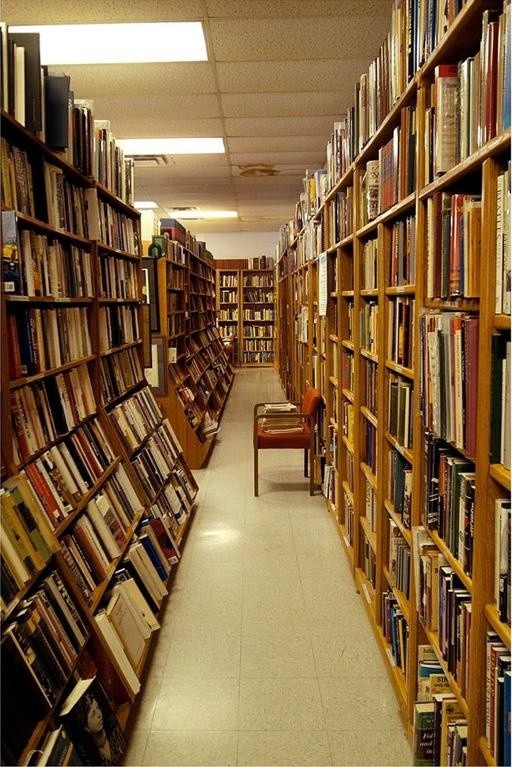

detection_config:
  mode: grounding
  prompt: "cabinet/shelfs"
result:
[277,0,512,766]
[142,236,235,468]
[0,107,199,763]
[239,268,276,367]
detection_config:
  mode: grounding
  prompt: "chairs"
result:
[252,387,322,497]
